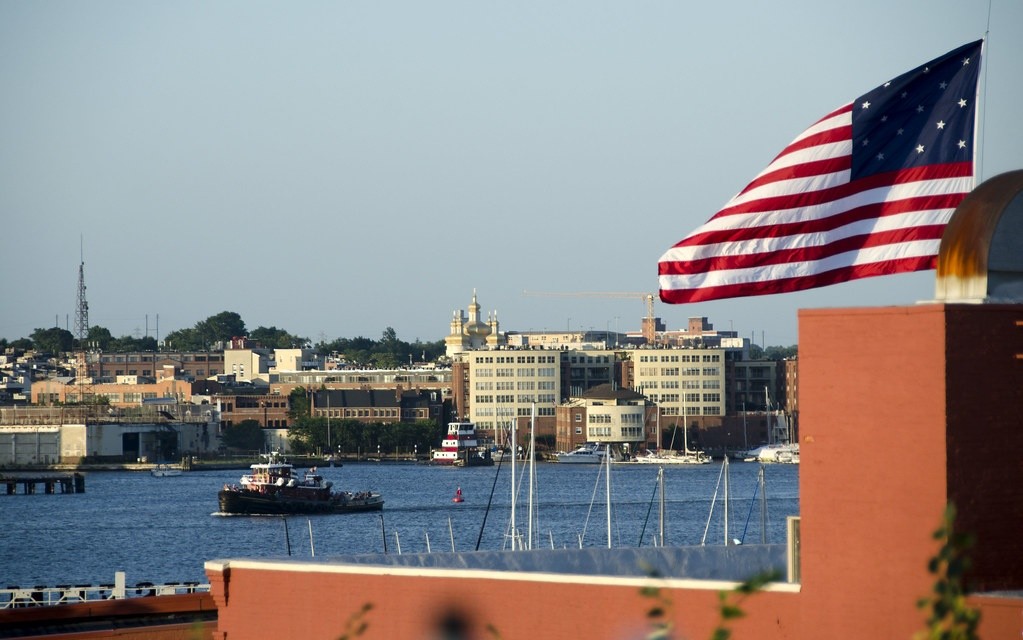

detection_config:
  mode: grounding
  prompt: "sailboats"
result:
[735,384,799,464]
[609,390,714,464]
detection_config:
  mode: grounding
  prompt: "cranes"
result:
[522,288,661,347]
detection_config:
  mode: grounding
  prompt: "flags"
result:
[658,37,985,303]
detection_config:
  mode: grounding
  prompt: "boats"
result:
[0,569,218,638]
[429,416,496,467]
[557,445,606,463]
[217,431,385,517]
